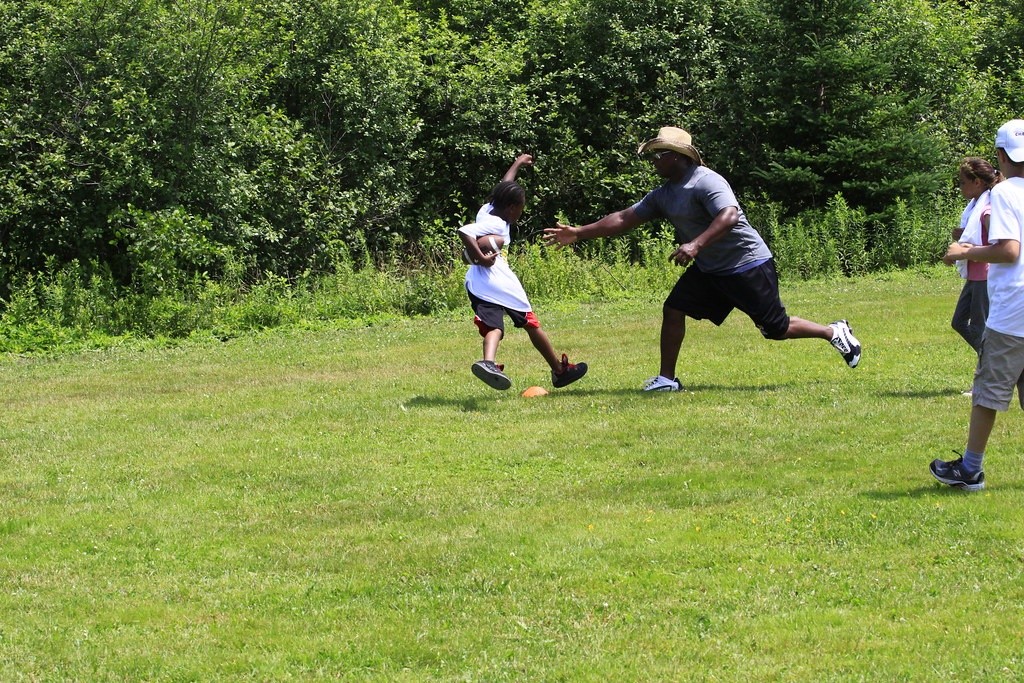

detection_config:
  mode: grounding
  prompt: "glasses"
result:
[994,148,999,155]
[653,149,671,160]
[960,178,973,184]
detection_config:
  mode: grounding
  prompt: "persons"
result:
[541,126,865,394]
[928,119,1024,497]
[456,151,587,391]
[944,156,1013,396]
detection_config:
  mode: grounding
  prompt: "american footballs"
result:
[461,234,504,266]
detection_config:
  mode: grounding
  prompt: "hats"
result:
[996,119,1024,162]
[638,126,706,167]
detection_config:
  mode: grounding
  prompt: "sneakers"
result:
[929,458,986,491]
[828,319,860,368]
[551,353,588,388]
[472,361,511,390]
[644,377,683,391]
